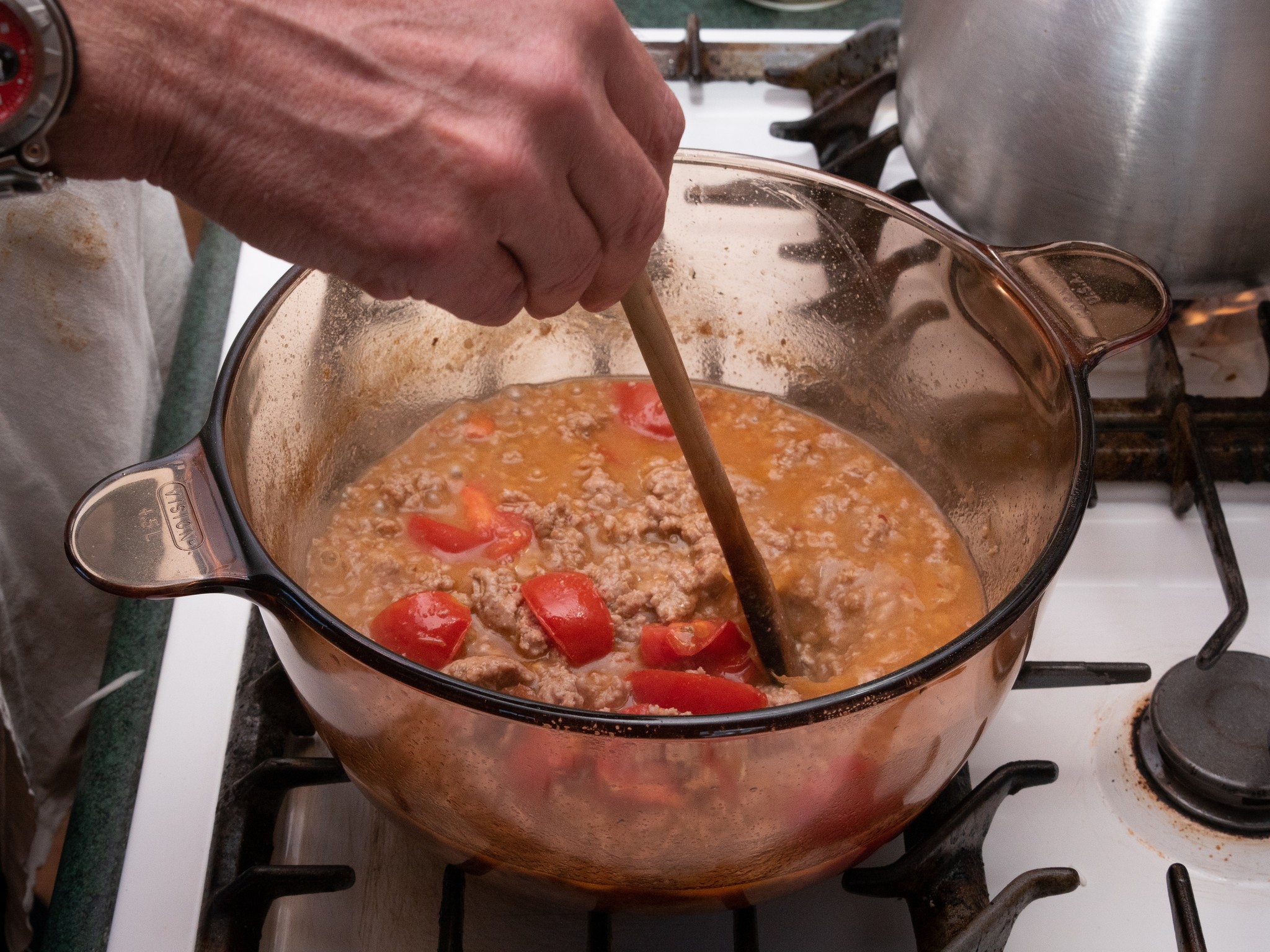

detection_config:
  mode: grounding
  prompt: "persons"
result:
[0,0,685,952]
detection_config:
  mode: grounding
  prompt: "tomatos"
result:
[371,488,901,847]
[616,383,677,437]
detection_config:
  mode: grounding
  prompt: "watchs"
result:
[0,0,81,199]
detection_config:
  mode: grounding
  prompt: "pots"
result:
[893,0,1270,306]
[65,147,1174,895]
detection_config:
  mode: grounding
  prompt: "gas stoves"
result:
[191,11,1270,952]
